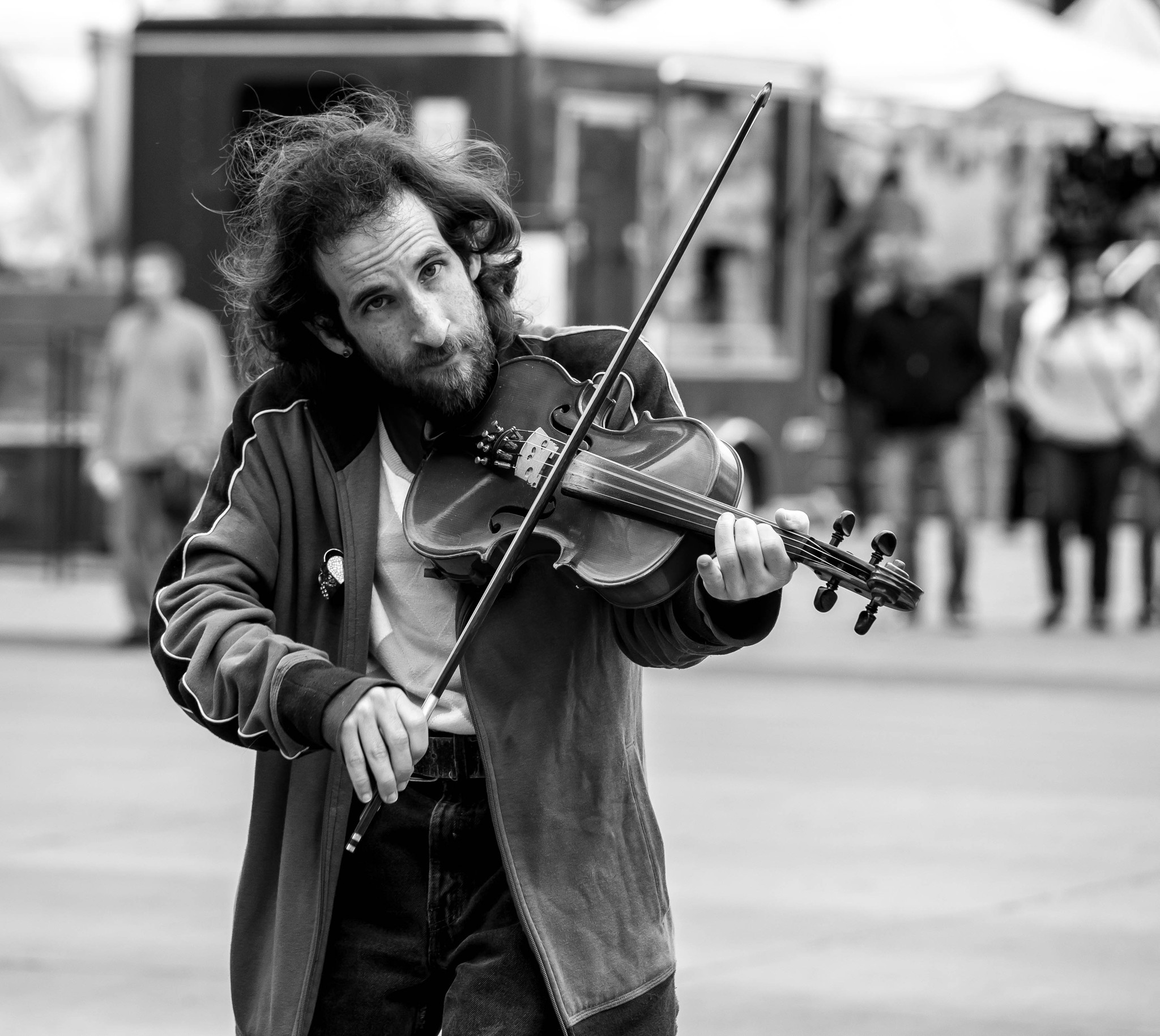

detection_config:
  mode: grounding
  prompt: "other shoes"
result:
[1033,600,1068,630]
[1087,603,1109,640]
[1135,600,1157,632]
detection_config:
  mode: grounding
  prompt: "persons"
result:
[805,165,1159,635]
[81,240,240,650]
[148,89,811,1036]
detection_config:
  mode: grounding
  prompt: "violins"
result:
[401,354,926,637]
